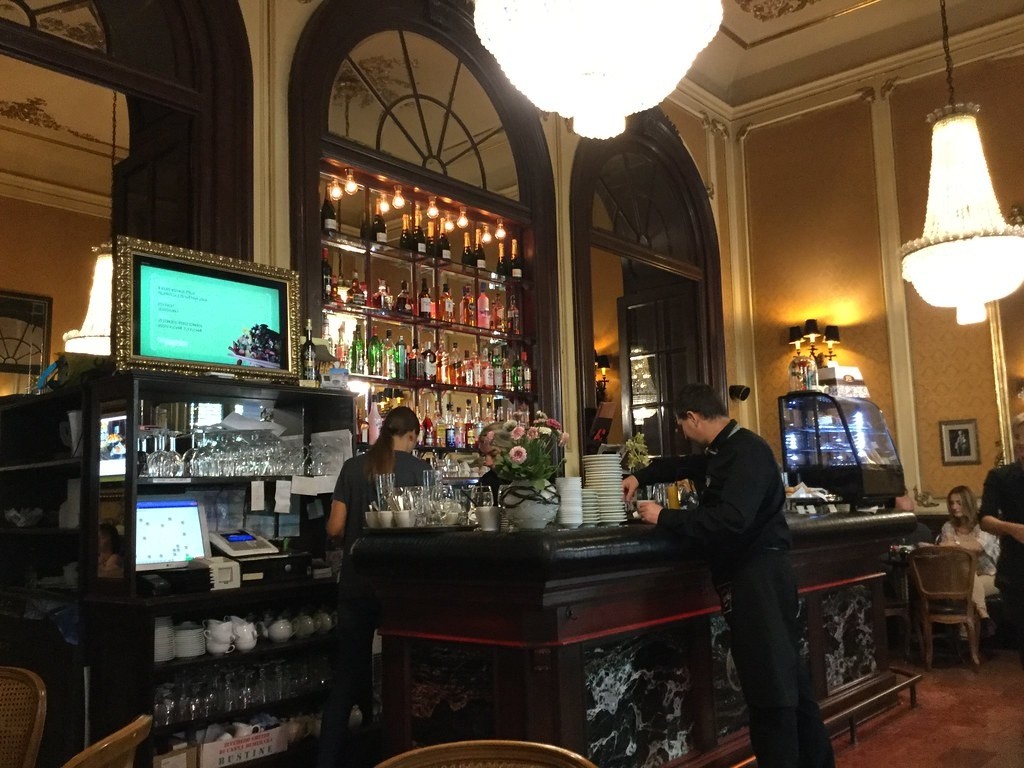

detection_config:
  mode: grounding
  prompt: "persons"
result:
[311,404,432,767]
[621,383,836,768]
[472,422,509,505]
[98,521,124,579]
[922,486,1002,664]
[979,413,1024,668]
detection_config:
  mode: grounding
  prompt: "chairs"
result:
[883,555,920,656]
[909,542,990,666]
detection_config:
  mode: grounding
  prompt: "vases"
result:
[501,479,561,529]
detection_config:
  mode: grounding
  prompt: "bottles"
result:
[302,180,531,448]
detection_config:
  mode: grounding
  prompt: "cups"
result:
[365,458,498,531]
[214,733,233,742]
[203,615,258,655]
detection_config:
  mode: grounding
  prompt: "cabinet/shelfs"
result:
[0,381,85,593]
[86,581,386,768]
[320,171,532,452]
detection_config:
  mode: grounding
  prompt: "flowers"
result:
[484,409,569,491]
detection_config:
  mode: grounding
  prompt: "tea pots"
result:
[256,610,339,643]
[276,705,362,744]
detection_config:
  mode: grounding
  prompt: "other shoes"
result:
[979,616,996,638]
[960,641,970,656]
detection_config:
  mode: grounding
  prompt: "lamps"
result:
[63,90,116,356]
[595,349,609,403]
[789,319,841,367]
[472,1,725,140]
[897,1,1024,325]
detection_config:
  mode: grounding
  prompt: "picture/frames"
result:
[939,418,981,465]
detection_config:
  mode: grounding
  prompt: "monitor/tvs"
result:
[134,494,212,571]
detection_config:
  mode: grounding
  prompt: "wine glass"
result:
[134,427,332,476]
[150,654,333,727]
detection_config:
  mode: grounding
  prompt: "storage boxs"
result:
[174,720,289,768]
[133,740,197,768]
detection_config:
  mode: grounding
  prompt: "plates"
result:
[154,615,205,663]
[554,453,628,528]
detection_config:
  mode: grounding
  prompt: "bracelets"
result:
[955,540,960,548]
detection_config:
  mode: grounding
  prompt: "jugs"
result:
[232,720,260,738]
